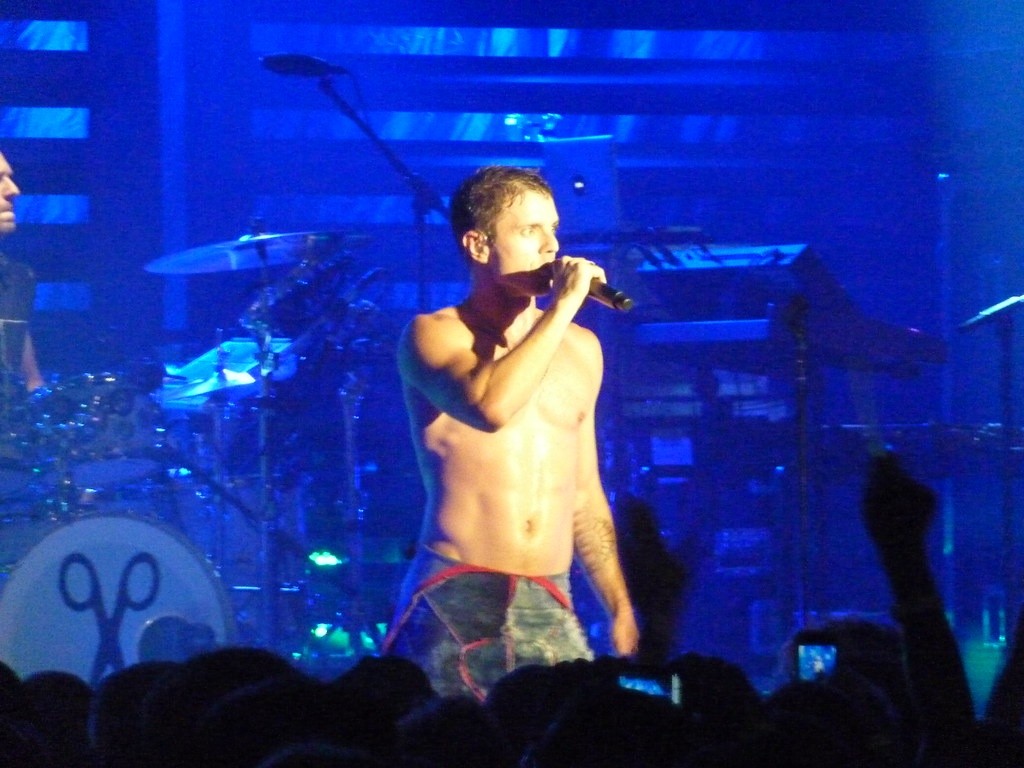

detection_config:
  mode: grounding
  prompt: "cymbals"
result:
[144,228,352,276]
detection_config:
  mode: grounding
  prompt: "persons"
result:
[0,450,1024,768]
[386,166,641,701]
[0,153,54,402]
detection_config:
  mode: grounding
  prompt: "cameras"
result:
[794,642,841,685]
[616,670,688,708]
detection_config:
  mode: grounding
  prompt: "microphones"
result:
[260,53,347,78]
[586,278,632,312]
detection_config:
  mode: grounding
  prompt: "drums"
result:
[0,507,238,688]
[176,472,307,593]
[0,366,33,499]
[21,371,167,494]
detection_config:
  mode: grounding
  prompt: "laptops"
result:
[542,135,701,232]
